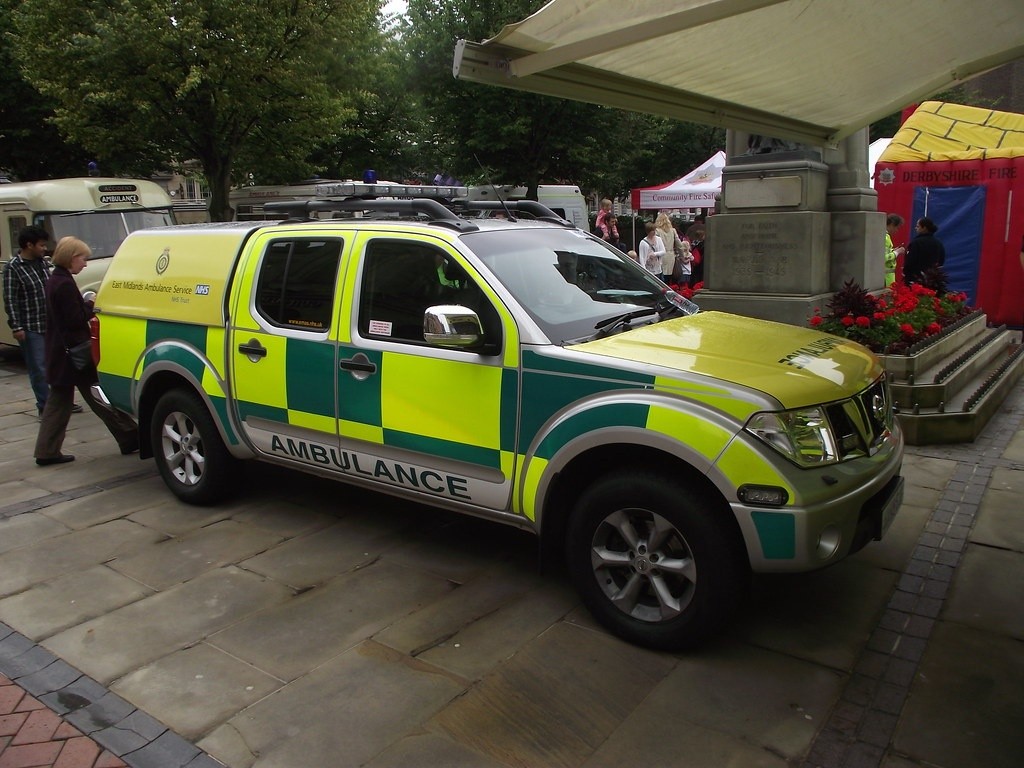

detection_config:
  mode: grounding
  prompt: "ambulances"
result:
[224,168,590,234]
[90,200,906,650]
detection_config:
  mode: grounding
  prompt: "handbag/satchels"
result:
[671,257,683,280]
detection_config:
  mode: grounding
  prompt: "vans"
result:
[1,177,177,356]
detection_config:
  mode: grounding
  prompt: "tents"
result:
[631,150,727,251]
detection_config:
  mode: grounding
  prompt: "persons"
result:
[3,226,84,423]
[902,218,945,288]
[495,211,514,219]
[594,198,619,250]
[34,236,138,466]
[654,213,706,289]
[627,222,666,282]
[885,214,905,287]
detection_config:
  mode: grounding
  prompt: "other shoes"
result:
[36,451,74,465]
[120,444,139,455]
[72,404,83,413]
[37,414,42,422]
